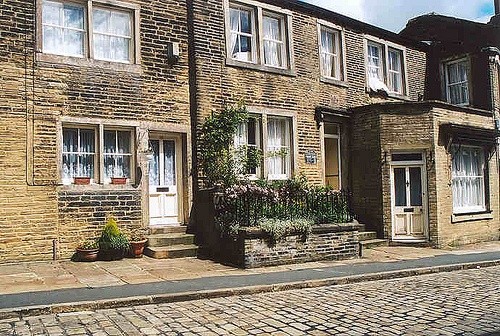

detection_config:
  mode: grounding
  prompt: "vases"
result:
[72,177,92,184]
[99,248,128,261]
[110,177,128,183]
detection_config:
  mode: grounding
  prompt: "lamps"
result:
[167,42,181,60]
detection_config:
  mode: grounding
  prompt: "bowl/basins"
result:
[111,177,127,184]
[73,177,90,185]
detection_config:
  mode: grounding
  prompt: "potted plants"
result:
[140,148,155,161]
[76,242,100,262]
[127,230,148,258]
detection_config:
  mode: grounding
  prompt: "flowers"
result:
[99,216,131,249]
